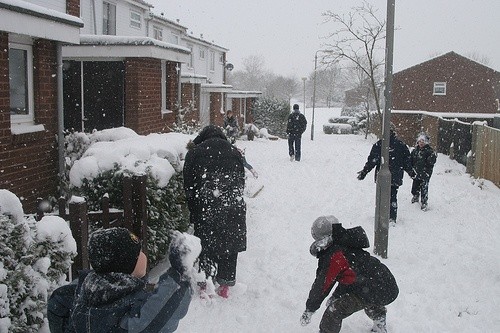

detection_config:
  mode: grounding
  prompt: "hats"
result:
[87,227,142,275]
[390,121,396,131]
[310,214,339,241]
[293,104,299,110]
[416,134,429,144]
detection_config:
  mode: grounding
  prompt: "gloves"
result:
[300,310,313,325]
[169,236,191,271]
[357,166,369,180]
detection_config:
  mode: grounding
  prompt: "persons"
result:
[242,156,258,179]
[64,227,194,333]
[183,125,247,298]
[356,122,417,228]
[285,104,307,162]
[223,110,238,143]
[410,132,438,211]
[299,215,399,333]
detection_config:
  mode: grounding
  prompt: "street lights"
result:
[301,77,308,117]
[311,49,335,141]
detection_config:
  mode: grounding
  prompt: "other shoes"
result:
[370,320,387,333]
[199,287,212,302]
[411,197,419,203]
[421,203,428,211]
[290,156,294,160]
[215,284,229,299]
